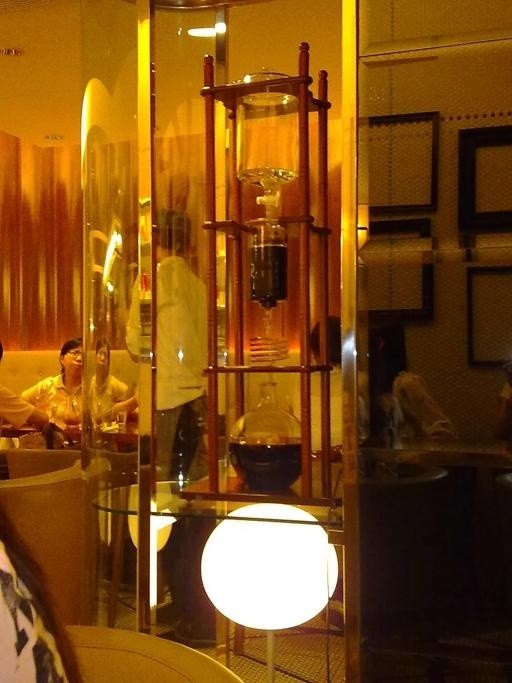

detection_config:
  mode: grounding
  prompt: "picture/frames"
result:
[356,109,439,216]
[466,264,511,367]
[359,218,433,324]
[457,125,511,232]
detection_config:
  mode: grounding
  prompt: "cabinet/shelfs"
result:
[180,38,339,505]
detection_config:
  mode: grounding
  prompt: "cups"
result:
[116,408,128,425]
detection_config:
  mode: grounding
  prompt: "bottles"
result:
[245,216,290,304]
[234,73,299,223]
[228,382,303,492]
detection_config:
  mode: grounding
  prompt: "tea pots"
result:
[63,394,82,428]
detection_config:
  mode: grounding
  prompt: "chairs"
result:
[2,424,245,480]
[2,459,105,672]
[64,622,246,681]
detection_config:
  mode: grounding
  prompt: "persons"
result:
[290,311,368,504]
[367,316,458,448]
[18,338,86,426]
[0,340,51,430]
[85,338,134,425]
[111,206,219,478]
